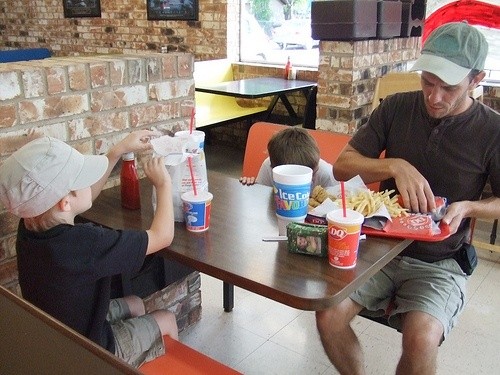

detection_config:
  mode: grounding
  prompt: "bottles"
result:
[285,57,292,80]
[120,152,140,210]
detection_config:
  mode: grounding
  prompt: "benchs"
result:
[242,120,386,193]
[0,286,245,375]
[192,59,267,129]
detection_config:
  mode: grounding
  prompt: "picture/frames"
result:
[147,0,198,20]
[63,0,101,18]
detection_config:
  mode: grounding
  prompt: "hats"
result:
[408,19,488,85]
[1,136,108,218]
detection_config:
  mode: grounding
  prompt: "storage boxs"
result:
[310,0,427,41]
[286,220,328,256]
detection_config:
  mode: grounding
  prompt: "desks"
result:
[195,77,317,126]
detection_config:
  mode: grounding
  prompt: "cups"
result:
[326,209,364,270]
[181,190,214,232]
[272,164,314,236]
[288,67,297,80]
[173,130,205,152]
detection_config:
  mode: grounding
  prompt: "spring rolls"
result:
[307,186,337,210]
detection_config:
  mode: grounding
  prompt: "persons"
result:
[316,20,500,375]
[239,128,341,204]
[0,136,178,370]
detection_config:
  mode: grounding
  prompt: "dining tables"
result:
[75,168,414,312]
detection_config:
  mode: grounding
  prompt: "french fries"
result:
[339,189,409,217]
